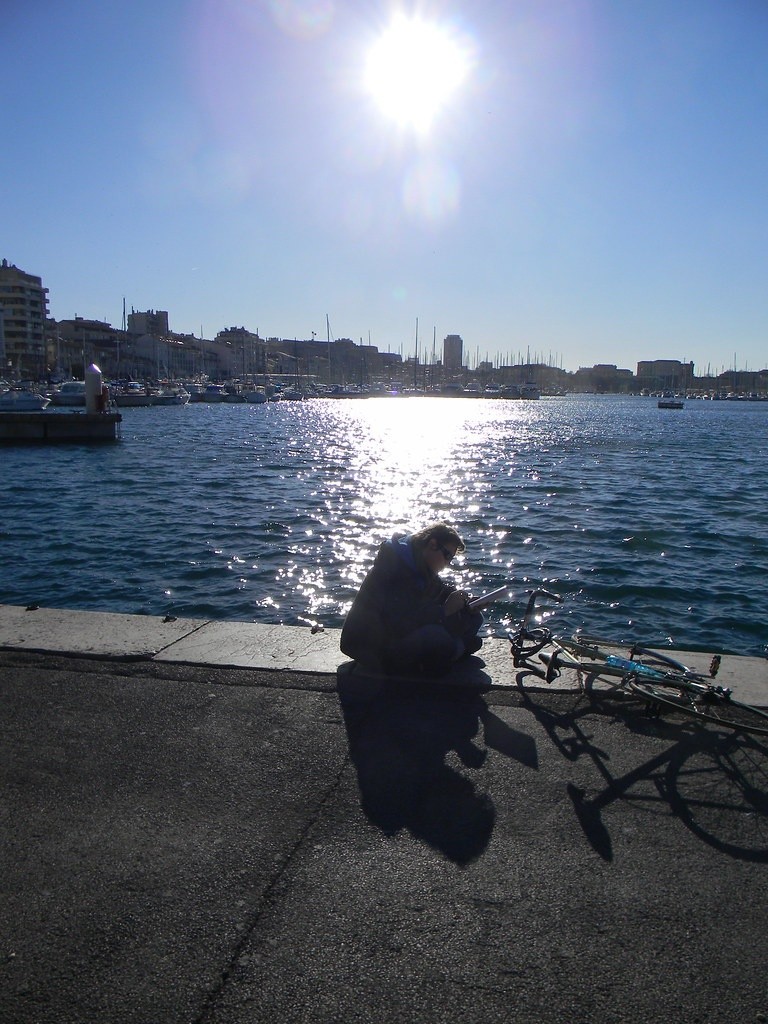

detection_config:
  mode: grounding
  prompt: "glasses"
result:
[437,543,454,562]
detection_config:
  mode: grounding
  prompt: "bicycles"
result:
[512,586,768,773]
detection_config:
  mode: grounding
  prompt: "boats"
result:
[54,388,191,406]
[188,383,303,405]
[640,388,768,401]
[544,388,567,397]
[0,388,51,411]
[463,382,541,400]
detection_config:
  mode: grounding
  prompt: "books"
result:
[468,586,509,610]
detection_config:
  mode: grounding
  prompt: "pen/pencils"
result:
[460,592,470,605]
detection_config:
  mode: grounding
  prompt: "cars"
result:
[276,379,441,393]
[1,373,243,390]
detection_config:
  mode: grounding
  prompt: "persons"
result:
[340,524,493,680]
